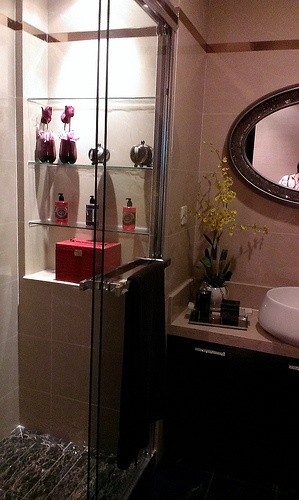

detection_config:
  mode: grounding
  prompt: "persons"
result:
[278,161,299,190]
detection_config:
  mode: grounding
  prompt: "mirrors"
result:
[225,81,299,210]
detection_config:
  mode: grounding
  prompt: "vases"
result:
[199,278,231,308]
[37,138,56,164]
[58,139,77,165]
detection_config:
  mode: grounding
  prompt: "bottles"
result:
[130,140,154,168]
[89,144,110,163]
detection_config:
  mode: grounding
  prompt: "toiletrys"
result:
[121,197,137,231]
[53,192,69,224]
[85,195,100,226]
[194,281,212,322]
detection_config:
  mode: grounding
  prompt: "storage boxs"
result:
[55,237,121,285]
[219,298,240,322]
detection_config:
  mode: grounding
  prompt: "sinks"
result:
[257,285,299,348]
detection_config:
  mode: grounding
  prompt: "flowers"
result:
[59,105,80,141]
[179,139,271,287]
[36,106,55,143]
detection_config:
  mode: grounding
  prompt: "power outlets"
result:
[180,205,188,226]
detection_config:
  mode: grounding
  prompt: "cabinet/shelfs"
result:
[167,335,299,486]
[27,96,156,237]
